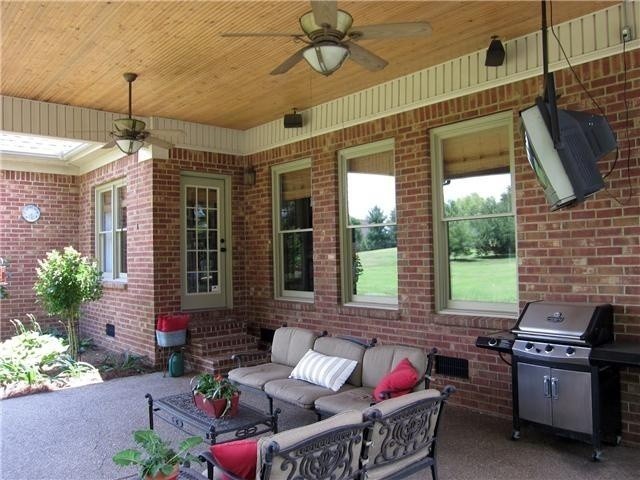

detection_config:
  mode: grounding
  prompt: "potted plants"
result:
[115,428,204,480]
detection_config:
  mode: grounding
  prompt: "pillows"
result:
[207,437,260,479]
[288,348,358,393]
[372,355,422,403]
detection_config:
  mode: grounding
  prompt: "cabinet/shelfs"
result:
[512,356,619,460]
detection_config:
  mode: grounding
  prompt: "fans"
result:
[70,73,186,149]
[223,0,433,74]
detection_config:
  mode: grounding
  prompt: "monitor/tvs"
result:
[519,96,618,213]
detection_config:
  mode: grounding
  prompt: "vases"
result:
[193,392,239,418]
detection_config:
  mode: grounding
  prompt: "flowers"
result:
[188,375,241,418]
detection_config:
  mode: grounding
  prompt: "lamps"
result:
[300,44,350,74]
[115,138,144,154]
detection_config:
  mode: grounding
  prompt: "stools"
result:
[161,346,194,377]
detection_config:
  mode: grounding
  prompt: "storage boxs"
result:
[155,329,187,347]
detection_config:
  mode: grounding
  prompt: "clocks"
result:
[22,205,40,222]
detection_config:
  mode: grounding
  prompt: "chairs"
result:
[315,338,440,425]
[226,322,328,411]
[359,384,455,479]
[265,330,381,432]
[200,410,369,479]
